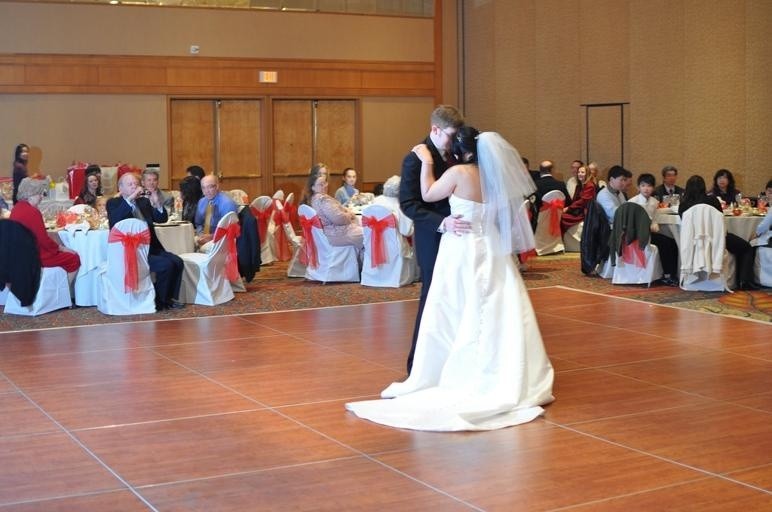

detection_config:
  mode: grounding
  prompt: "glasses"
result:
[314,181,329,186]
[433,122,456,142]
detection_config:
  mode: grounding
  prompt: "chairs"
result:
[297,204,361,284]
[611,202,663,285]
[284,193,295,223]
[362,204,421,287]
[223,205,256,293]
[563,221,583,252]
[535,190,565,255]
[275,200,307,280]
[91,218,158,316]
[0,222,71,313]
[179,211,238,305]
[752,245,772,291]
[680,203,736,292]
[265,187,284,261]
[247,195,273,266]
[590,201,621,279]
[509,200,532,270]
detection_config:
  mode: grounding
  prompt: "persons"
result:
[346,128,555,431]
[624,169,633,200]
[650,166,687,203]
[308,175,366,272]
[12,177,80,291]
[709,169,743,204]
[73,165,106,213]
[750,201,772,259]
[521,158,540,183]
[561,165,596,236]
[595,164,628,229]
[566,160,584,200]
[11,144,28,203]
[757,180,772,204]
[532,160,571,231]
[194,176,237,254]
[627,174,679,287]
[375,176,414,237]
[179,166,205,223]
[399,107,471,376]
[137,169,175,214]
[336,169,360,206]
[105,173,184,312]
[302,164,328,205]
[678,175,760,290]
[588,162,608,190]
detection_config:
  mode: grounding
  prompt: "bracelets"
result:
[422,161,436,166]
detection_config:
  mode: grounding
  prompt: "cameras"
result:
[140,188,152,196]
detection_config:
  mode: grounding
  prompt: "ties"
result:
[133,199,146,221]
[204,200,214,235]
